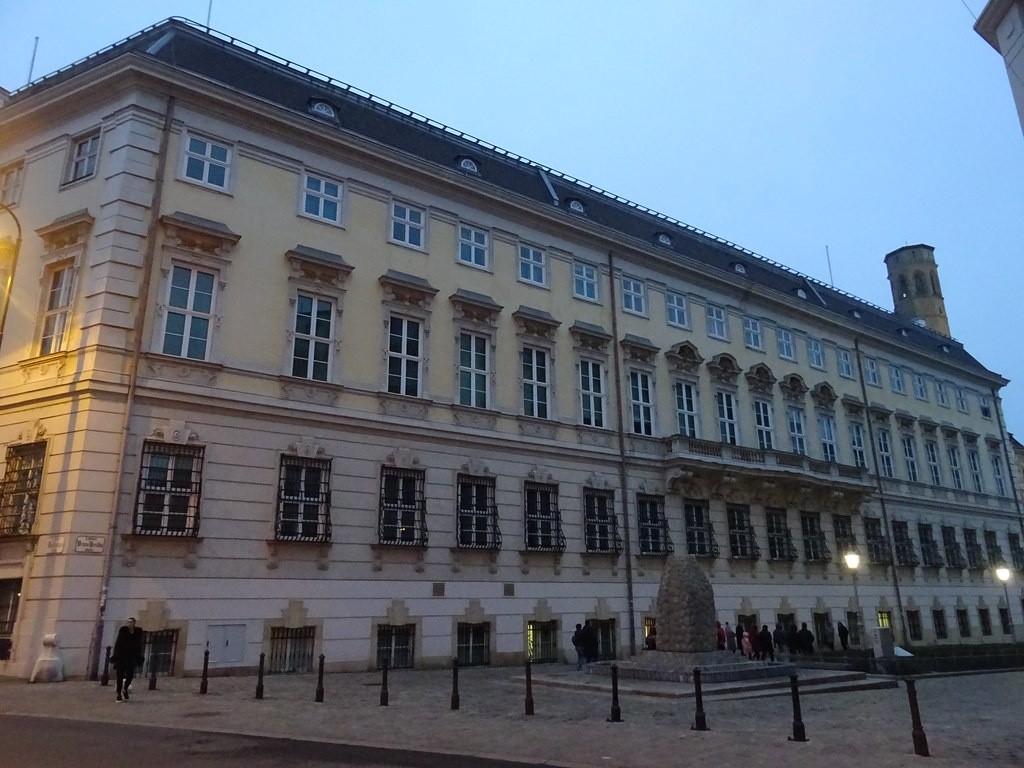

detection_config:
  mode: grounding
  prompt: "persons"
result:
[646,631,656,650]
[824,620,835,652]
[716,619,813,660]
[838,622,849,651]
[571,620,599,672]
[110,619,146,702]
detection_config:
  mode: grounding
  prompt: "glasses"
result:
[127,621,133,623]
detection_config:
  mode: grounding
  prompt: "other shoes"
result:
[116,694,123,703]
[122,689,129,700]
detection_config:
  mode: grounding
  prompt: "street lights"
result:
[996,563,1017,651]
[842,545,865,649]
